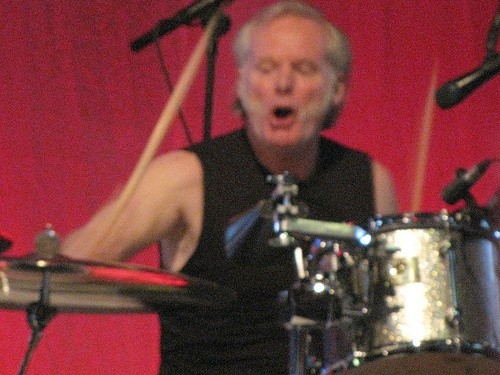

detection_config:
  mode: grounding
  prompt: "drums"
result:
[289,210,500,375]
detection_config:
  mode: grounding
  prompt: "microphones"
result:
[442,161,490,205]
[435,53,500,109]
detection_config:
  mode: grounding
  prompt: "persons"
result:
[61,1,399,375]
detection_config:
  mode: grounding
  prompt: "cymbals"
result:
[0,248,240,315]
